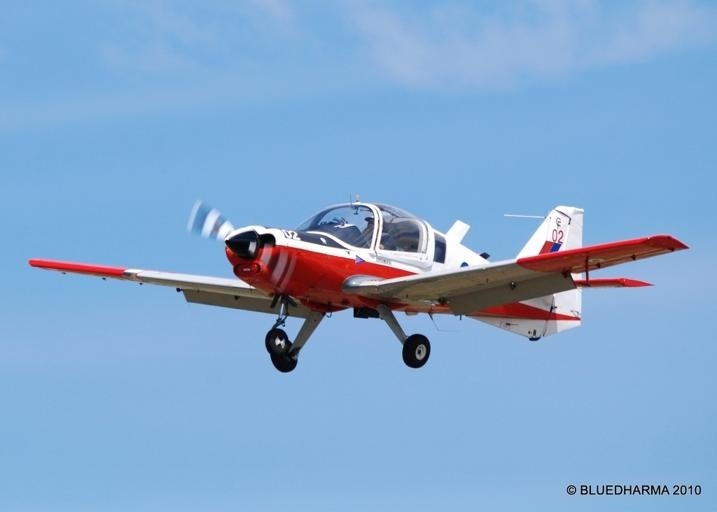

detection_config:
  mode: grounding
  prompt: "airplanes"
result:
[27,196,690,370]
